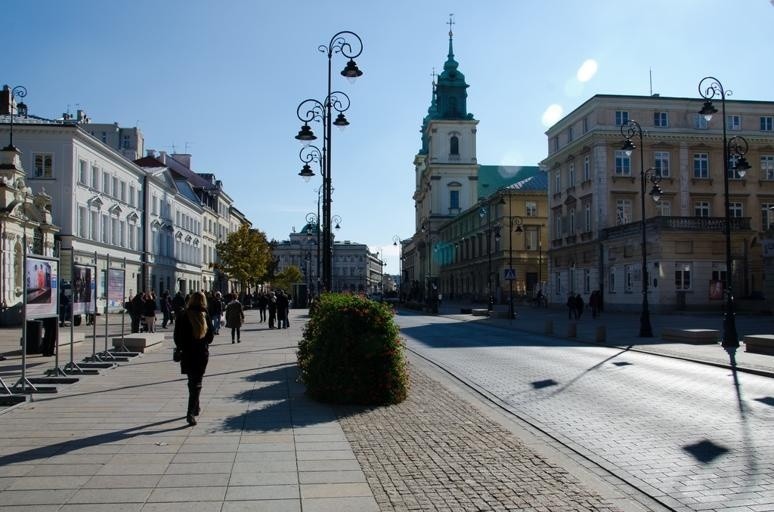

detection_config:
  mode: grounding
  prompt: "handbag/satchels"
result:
[174,347,182,362]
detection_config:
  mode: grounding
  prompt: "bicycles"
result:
[531,296,548,308]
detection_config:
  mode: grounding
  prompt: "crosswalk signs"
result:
[504,269,516,281]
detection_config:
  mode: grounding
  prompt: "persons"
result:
[567,288,601,320]
[126,288,291,344]
[173,290,214,426]
[27,263,45,288]
[60,288,70,327]
[536,289,544,307]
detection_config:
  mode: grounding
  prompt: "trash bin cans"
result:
[20,320,43,354]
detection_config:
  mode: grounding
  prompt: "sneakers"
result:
[186,414,198,425]
[194,408,200,416]
[232,339,241,344]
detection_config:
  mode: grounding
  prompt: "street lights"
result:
[697,76,753,366]
[495,186,523,320]
[392,234,406,308]
[294,91,352,306]
[478,200,500,313]
[421,219,438,314]
[620,119,664,339]
[376,248,386,302]
[295,29,364,307]
